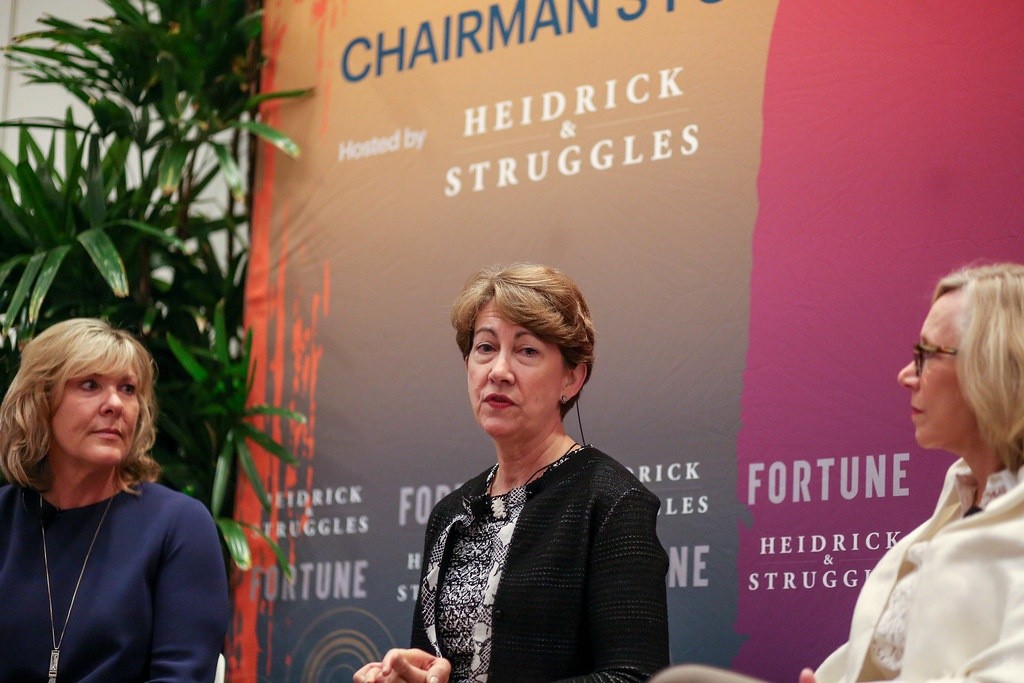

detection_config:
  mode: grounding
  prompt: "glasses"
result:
[913,344,958,375]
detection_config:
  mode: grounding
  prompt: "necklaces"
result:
[40,492,113,683]
[524,443,578,485]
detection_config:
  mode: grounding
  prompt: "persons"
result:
[353,263,669,683]
[800,262,1024,683]
[0,318,230,683]
